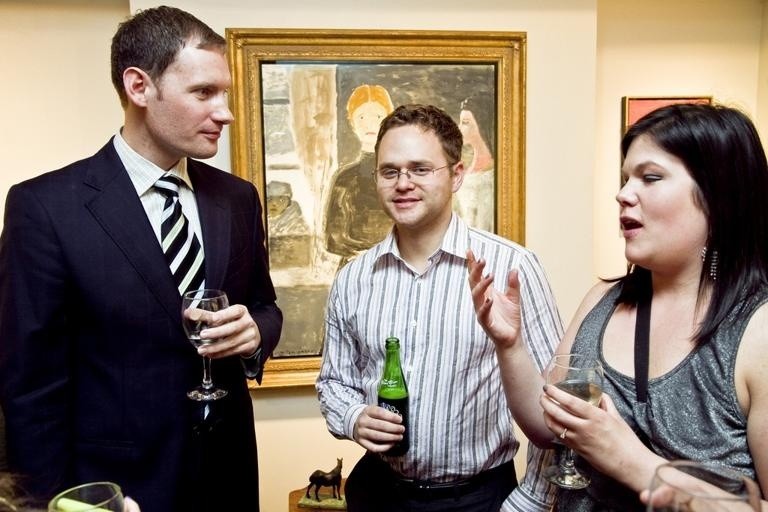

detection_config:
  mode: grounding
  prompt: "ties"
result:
[153,174,205,339]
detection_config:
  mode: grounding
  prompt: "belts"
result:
[367,456,510,496]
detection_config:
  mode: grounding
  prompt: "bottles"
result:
[377,337,410,456]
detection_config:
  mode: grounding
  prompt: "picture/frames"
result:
[224,26,528,390]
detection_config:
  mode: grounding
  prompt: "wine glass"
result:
[542,353,605,490]
[181,288,230,401]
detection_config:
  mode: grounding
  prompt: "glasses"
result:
[371,164,452,186]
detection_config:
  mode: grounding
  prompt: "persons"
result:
[0,5,283,512]
[450,91,495,232]
[324,84,394,263]
[265,180,308,269]
[465,103,768,512]
[316,104,565,512]
[640,481,758,512]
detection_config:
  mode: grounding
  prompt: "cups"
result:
[48,482,124,512]
[647,460,761,512]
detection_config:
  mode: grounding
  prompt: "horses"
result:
[306,457,343,502]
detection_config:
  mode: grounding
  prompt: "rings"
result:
[559,429,567,439]
